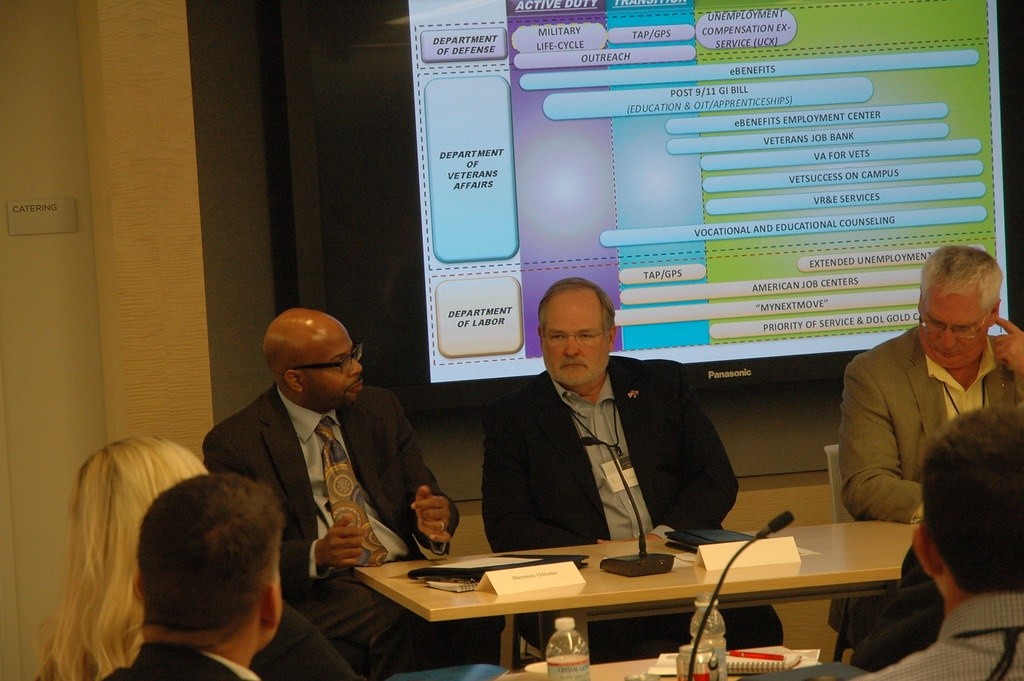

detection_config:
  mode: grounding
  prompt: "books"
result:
[403,552,590,592]
[643,643,824,673]
[656,526,759,556]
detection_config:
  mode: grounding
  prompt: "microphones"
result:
[688,510,794,681]
[580,436,676,576]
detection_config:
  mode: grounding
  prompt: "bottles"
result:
[691,594,727,681]
[546,617,591,681]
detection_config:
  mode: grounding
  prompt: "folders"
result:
[407,553,590,582]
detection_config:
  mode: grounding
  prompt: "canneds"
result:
[676,644,719,681]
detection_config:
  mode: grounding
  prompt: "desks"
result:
[354,519,919,661]
[589,645,822,680]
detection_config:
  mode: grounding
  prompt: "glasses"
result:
[920,298,994,339]
[543,330,609,347]
[285,342,363,374]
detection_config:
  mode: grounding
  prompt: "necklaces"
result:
[938,369,990,424]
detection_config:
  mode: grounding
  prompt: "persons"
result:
[480,276,784,664]
[204,305,517,680]
[27,433,361,681]
[99,472,291,681]
[846,405,1024,681]
[830,243,1024,649]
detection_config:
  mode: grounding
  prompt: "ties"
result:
[314,417,389,568]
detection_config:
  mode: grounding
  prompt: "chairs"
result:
[824,443,856,661]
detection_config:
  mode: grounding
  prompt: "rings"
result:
[438,520,446,531]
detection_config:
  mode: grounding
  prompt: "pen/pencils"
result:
[418,576,475,583]
[725,650,784,661]
[1000,362,1012,389]
